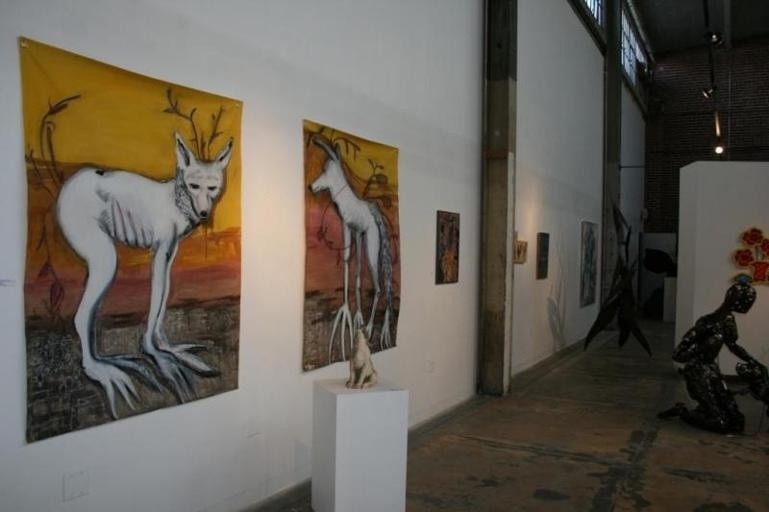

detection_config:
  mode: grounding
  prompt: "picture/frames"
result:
[536,232,550,280]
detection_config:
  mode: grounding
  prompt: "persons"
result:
[656,282,769,435]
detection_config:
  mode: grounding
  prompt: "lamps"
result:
[702,0,726,160]
